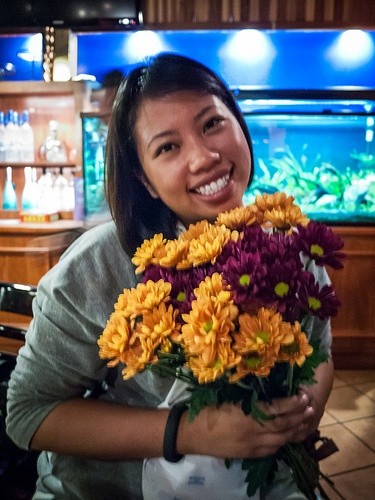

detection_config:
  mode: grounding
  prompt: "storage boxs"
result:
[78,88,375,232]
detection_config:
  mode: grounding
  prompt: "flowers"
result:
[97,191,348,499]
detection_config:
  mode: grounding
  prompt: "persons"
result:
[4,55,335,500]
[90,69,125,125]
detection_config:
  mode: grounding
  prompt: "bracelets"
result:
[163,404,189,463]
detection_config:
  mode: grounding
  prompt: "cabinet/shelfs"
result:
[0,80,91,356]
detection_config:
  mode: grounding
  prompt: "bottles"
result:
[3,166,18,210]
[39,120,67,164]
[21,167,77,214]
[0,109,35,164]
